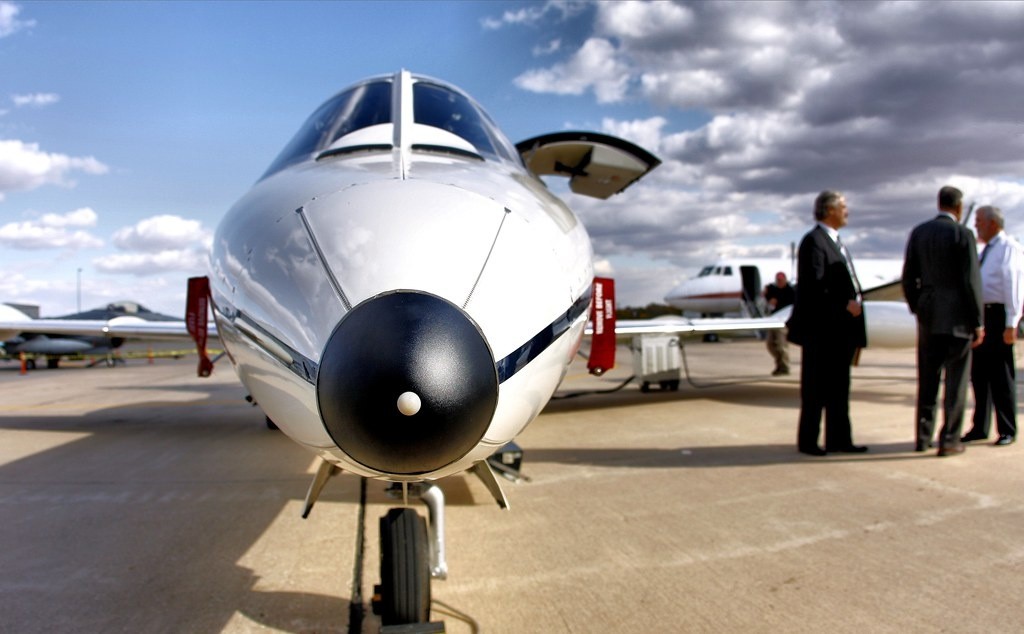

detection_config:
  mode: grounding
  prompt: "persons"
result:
[960,205,1024,446]
[764,273,795,375]
[902,186,985,456]
[791,189,869,456]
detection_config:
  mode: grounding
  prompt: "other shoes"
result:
[798,443,828,456]
[839,444,869,455]
[772,367,790,375]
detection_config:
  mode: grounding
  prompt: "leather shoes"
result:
[938,443,967,456]
[960,435,991,444]
[995,434,1014,446]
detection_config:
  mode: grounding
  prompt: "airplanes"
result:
[0,65,1023,634]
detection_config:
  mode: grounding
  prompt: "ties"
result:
[979,242,992,268]
